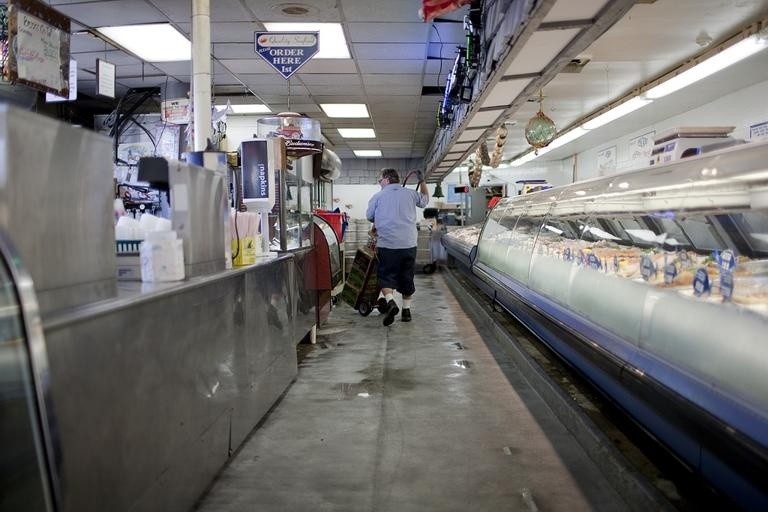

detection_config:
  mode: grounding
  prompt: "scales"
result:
[644,127,737,167]
[446,186,469,235]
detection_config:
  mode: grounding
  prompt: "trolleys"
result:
[341,171,422,317]
[415,224,437,274]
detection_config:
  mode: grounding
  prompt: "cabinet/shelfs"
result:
[269,138,318,256]
[470,132,767,469]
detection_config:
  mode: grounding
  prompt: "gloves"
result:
[416,169,424,182]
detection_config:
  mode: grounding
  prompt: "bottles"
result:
[139,229,186,282]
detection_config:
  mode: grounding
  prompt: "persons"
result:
[366,168,429,326]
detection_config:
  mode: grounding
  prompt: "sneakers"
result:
[402,309,411,322]
[383,300,399,326]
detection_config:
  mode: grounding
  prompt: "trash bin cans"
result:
[317,213,346,241]
